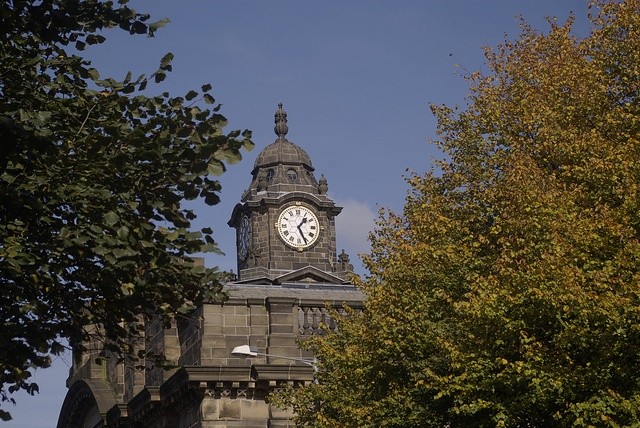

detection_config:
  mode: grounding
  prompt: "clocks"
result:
[237,216,251,262]
[274,200,325,252]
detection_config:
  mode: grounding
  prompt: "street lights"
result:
[230,343,319,412]
